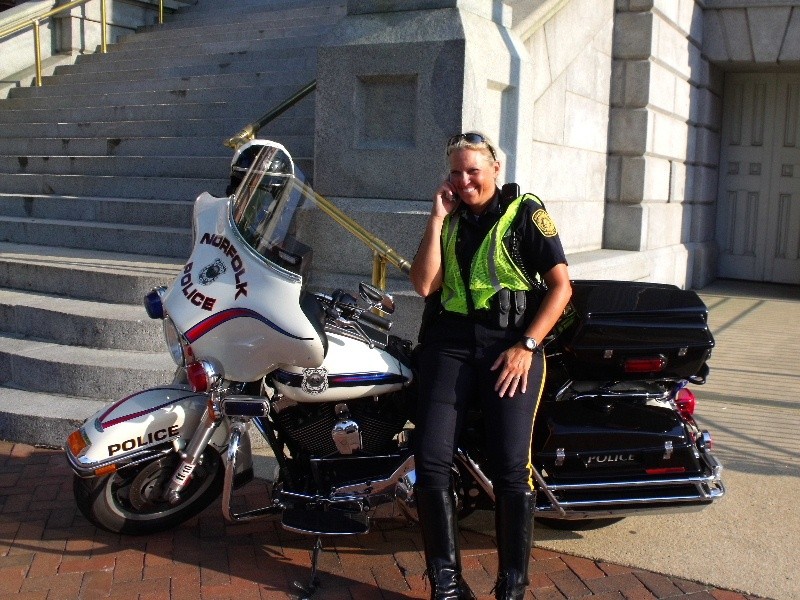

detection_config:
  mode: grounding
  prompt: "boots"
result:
[413,487,477,600]
[490,491,536,600]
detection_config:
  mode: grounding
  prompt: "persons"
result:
[410,130,572,600]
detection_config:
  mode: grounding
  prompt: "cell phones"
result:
[452,193,460,204]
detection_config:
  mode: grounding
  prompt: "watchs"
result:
[520,336,537,353]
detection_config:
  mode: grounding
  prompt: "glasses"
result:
[448,133,496,161]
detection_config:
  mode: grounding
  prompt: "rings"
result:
[515,378,520,379]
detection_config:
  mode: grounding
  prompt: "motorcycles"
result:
[62,138,726,600]
